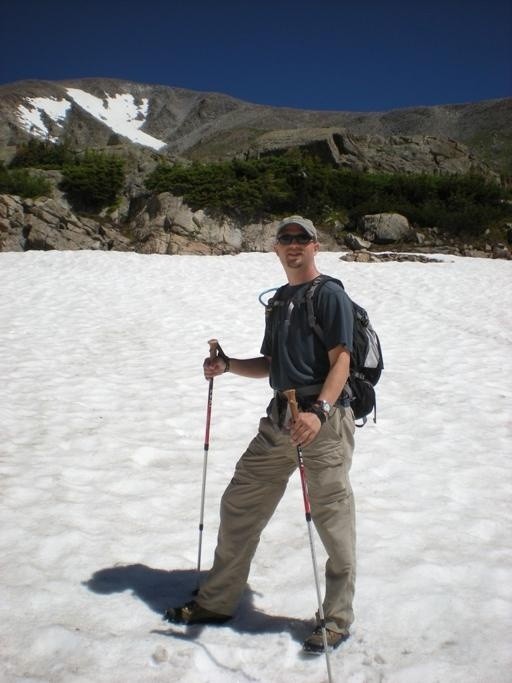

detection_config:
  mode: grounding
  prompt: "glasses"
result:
[279,233,312,245]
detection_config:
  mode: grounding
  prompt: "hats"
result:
[275,214,317,241]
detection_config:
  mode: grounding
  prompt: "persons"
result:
[160,213,359,651]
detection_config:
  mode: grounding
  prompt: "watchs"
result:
[310,396,332,415]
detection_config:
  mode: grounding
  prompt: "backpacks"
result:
[304,274,384,428]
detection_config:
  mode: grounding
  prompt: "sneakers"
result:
[165,599,233,625]
[303,625,344,655]
